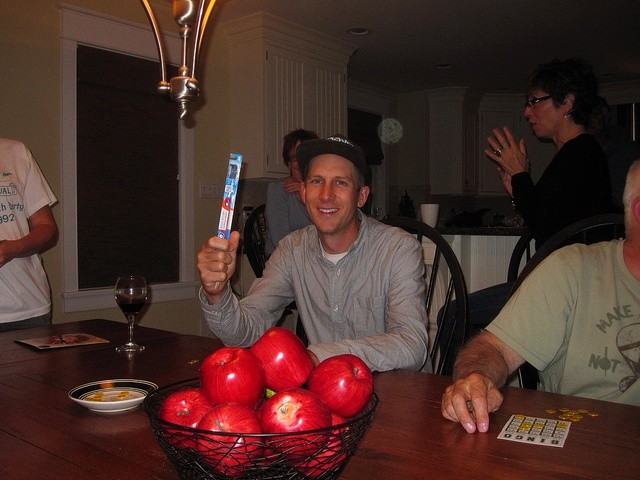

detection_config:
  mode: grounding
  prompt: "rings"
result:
[494,148,502,157]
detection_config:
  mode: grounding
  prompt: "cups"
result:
[420,204,440,228]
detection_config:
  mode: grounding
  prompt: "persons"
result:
[0,138,60,333]
[441,158,640,433]
[196,133,433,373]
[586,96,638,210]
[437,59,613,390]
[264,128,322,348]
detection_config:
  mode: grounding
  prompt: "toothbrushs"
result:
[218,163,239,239]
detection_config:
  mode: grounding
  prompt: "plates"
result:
[68,378,160,413]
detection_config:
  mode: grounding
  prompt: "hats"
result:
[297,134,368,179]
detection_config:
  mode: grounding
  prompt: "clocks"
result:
[377,118,405,145]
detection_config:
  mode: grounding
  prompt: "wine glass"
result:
[114,273,148,353]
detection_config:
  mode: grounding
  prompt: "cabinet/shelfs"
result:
[428,102,479,196]
[191,45,350,183]
[413,233,534,323]
[479,100,556,196]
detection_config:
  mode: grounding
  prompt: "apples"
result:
[195,400,263,475]
[259,387,333,460]
[251,407,281,465]
[157,386,213,449]
[285,413,351,476]
[199,346,267,411]
[309,354,373,418]
[249,326,315,393]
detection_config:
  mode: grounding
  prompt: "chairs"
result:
[296,216,470,377]
[507,224,543,284]
[505,224,630,390]
[244,203,271,281]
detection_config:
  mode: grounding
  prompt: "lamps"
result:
[139,1,221,124]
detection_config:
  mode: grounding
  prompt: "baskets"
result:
[143,377,379,479]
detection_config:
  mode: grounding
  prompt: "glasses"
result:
[524,94,556,108]
[288,154,297,161]
